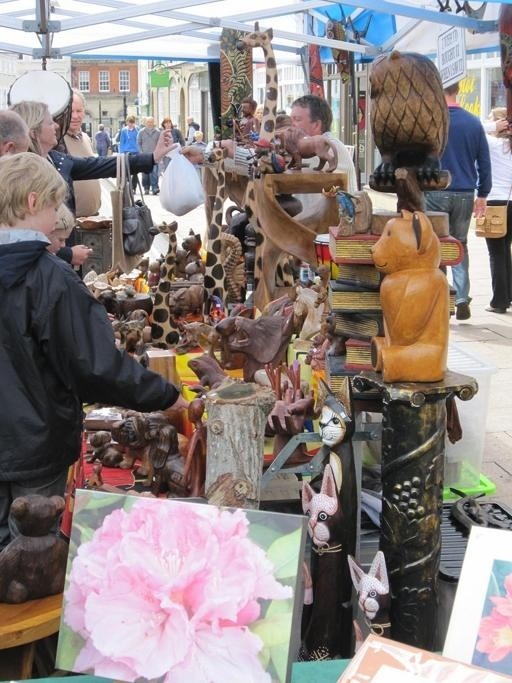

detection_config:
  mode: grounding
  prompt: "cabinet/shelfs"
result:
[76,227,112,279]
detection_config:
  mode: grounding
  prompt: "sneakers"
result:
[144,187,161,195]
[454,300,471,320]
[485,305,506,314]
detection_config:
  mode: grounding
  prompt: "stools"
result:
[0,591,63,679]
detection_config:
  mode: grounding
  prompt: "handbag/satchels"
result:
[121,149,155,256]
[474,204,509,239]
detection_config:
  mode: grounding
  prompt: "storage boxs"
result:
[361,340,500,489]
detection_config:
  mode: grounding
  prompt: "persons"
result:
[474,106,512,314]
[94,114,200,196]
[11,101,178,279]
[2,111,29,155]
[0,154,188,549]
[185,398,207,498]
[425,80,492,319]
[45,202,74,255]
[237,99,260,145]
[56,92,101,217]
[179,95,358,222]
